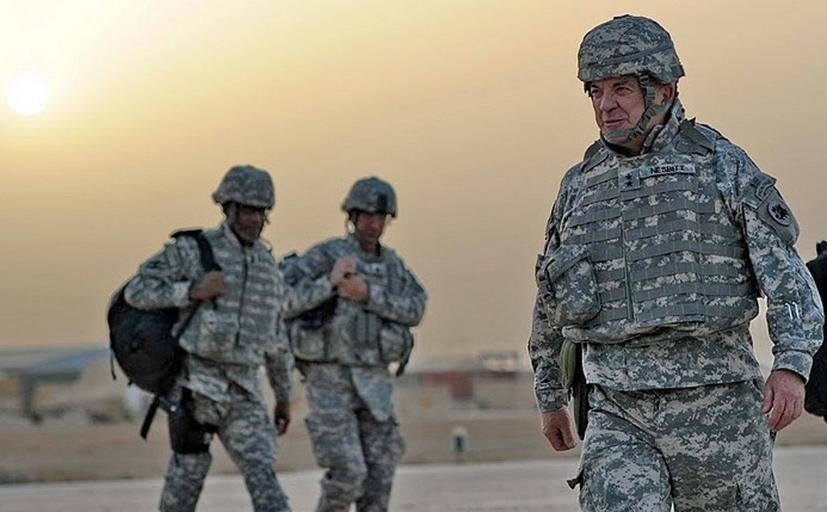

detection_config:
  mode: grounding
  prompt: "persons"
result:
[279,176,427,512]
[124,164,295,512]
[527,14,826,512]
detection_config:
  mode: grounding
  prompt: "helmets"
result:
[213,166,274,212]
[341,176,398,218]
[578,15,684,85]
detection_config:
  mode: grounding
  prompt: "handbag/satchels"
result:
[107,230,218,400]
[799,240,827,424]
